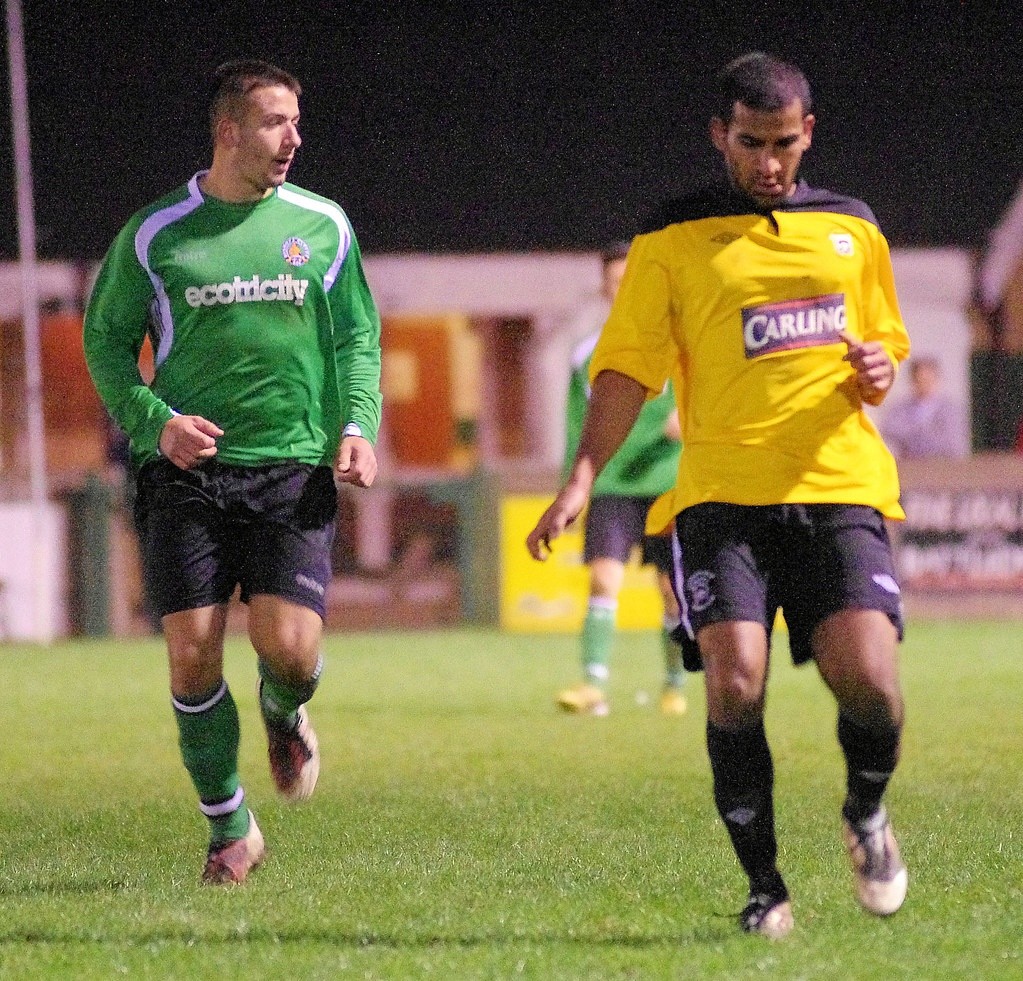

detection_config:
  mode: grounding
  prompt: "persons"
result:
[878,356,970,459]
[554,240,684,714]
[528,53,909,932]
[83,60,382,885]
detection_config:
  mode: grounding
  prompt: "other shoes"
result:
[660,688,686,713]
[557,682,609,716]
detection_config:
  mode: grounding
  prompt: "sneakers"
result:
[712,894,796,938]
[202,809,266,885]
[840,801,909,915]
[256,677,320,801]
